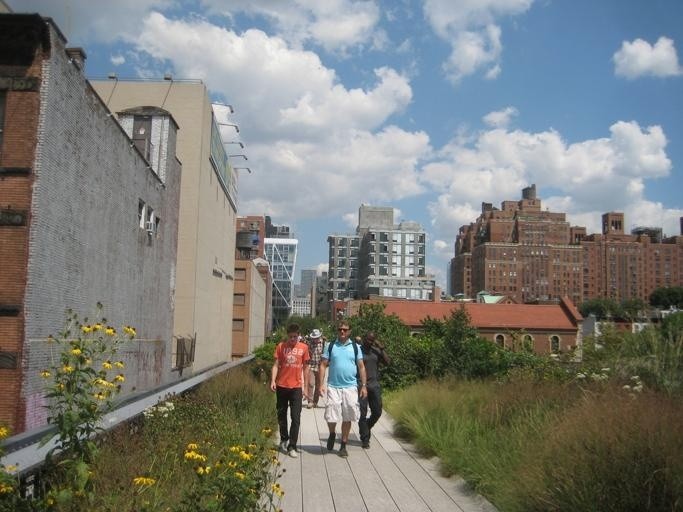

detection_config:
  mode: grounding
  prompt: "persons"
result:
[318,319,369,456]
[268,324,310,456]
[355,332,390,448]
[305,328,325,408]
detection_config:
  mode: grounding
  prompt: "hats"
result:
[310,329,322,339]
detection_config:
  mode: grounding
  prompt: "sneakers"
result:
[327,433,336,450]
[280,441,297,458]
[340,448,347,456]
[307,401,318,408]
[362,441,370,449]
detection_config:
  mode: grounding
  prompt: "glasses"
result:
[337,328,350,332]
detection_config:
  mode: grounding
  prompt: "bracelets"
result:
[380,348,384,350]
[360,383,367,387]
